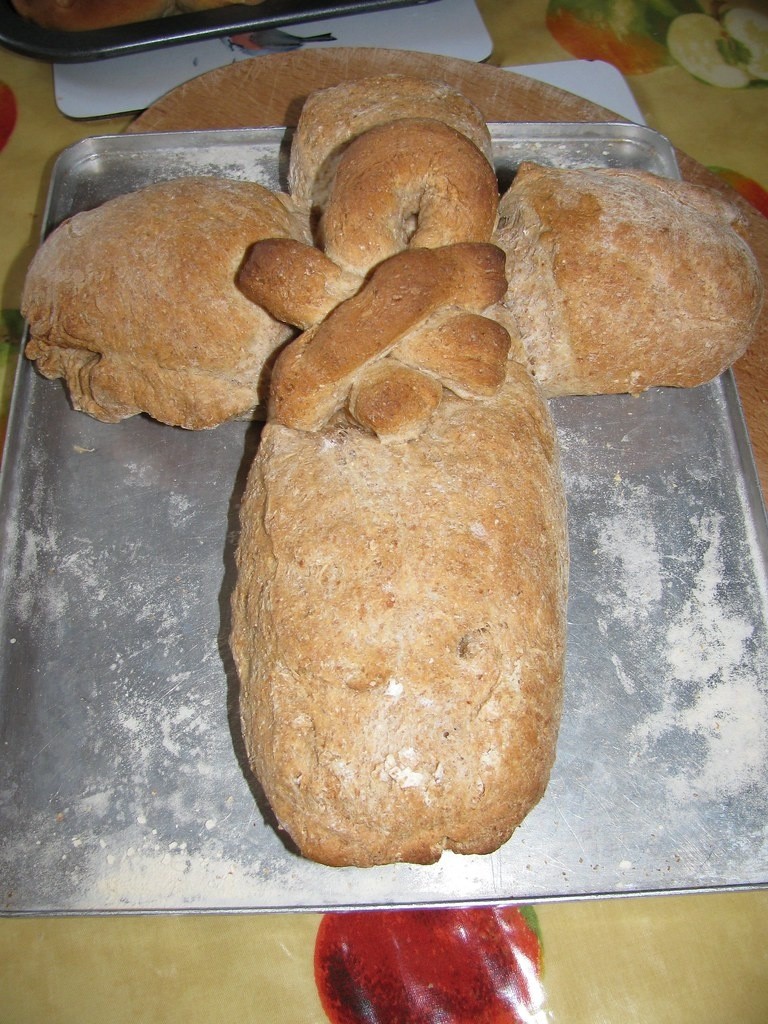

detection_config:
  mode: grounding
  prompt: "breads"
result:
[19,73,764,866]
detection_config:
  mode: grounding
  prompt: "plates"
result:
[0,122,768,915]
[0,0,441,64]
